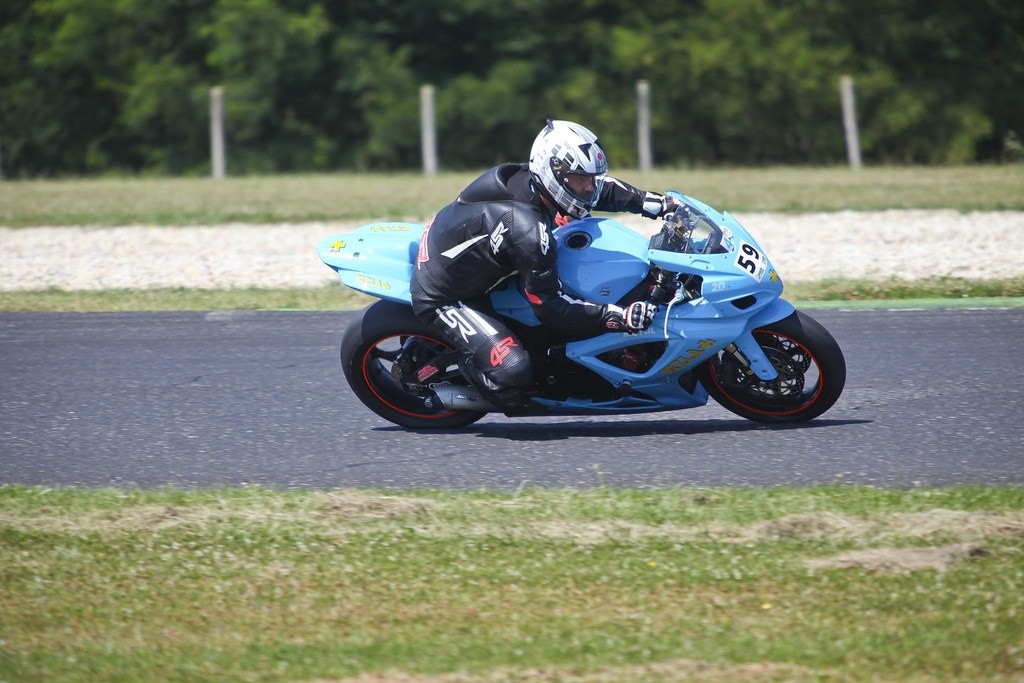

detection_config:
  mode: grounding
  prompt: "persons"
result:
[411,120,691,407]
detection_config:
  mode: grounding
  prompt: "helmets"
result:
[528,118,608,219]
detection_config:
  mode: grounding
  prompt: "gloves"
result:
[602,301,660,332]
[641,190,691,221]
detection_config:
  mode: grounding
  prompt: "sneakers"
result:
[455,352,520,406]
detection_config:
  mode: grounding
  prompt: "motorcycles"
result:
[316,192,847,434]
